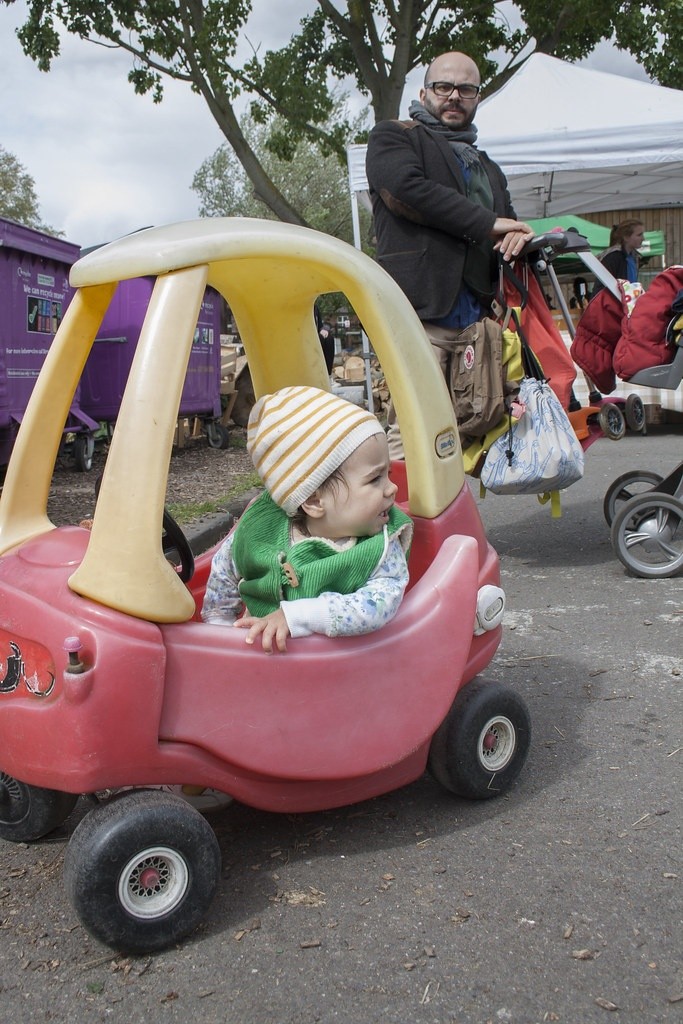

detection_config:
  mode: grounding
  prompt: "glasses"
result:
[425,81,482,99]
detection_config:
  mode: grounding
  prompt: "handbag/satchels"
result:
[480,378,585,495]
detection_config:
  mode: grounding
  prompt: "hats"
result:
[246,386,384,516]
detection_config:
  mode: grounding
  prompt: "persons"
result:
[200,386,414,656]
[366,51,538,460]
[589,219,646,301]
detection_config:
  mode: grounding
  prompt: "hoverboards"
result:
[537,251,645,452]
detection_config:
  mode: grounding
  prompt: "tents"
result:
[523,216,666,271]
[346,51,683,415]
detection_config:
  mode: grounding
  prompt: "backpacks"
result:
[430,299,562,518]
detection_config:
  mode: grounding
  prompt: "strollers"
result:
[517,230,683,580]
